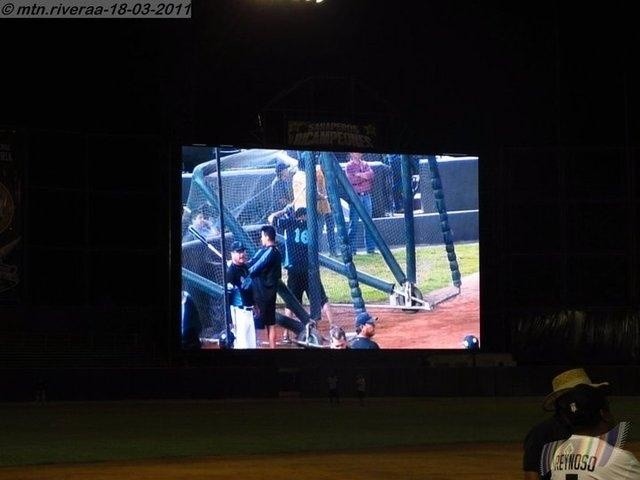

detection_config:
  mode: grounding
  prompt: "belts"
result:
[239,305,253,312]
[358,192,370,196]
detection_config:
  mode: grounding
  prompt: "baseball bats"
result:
[188,225,229,265]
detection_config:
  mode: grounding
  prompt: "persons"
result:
[32,372,48,408]
[182,151,421,349]
[460,334,479,349]
[538,384,639,480]
[324,368,369,409]
[522,367,611,480]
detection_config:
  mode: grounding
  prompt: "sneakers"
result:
[282,334,292,343]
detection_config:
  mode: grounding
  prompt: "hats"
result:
[465,336,479,349]
[543,367,610,411]
[356,313,378,323]
[219,330,235,344]
[276,162,290,173]
[230,241,249,252]
